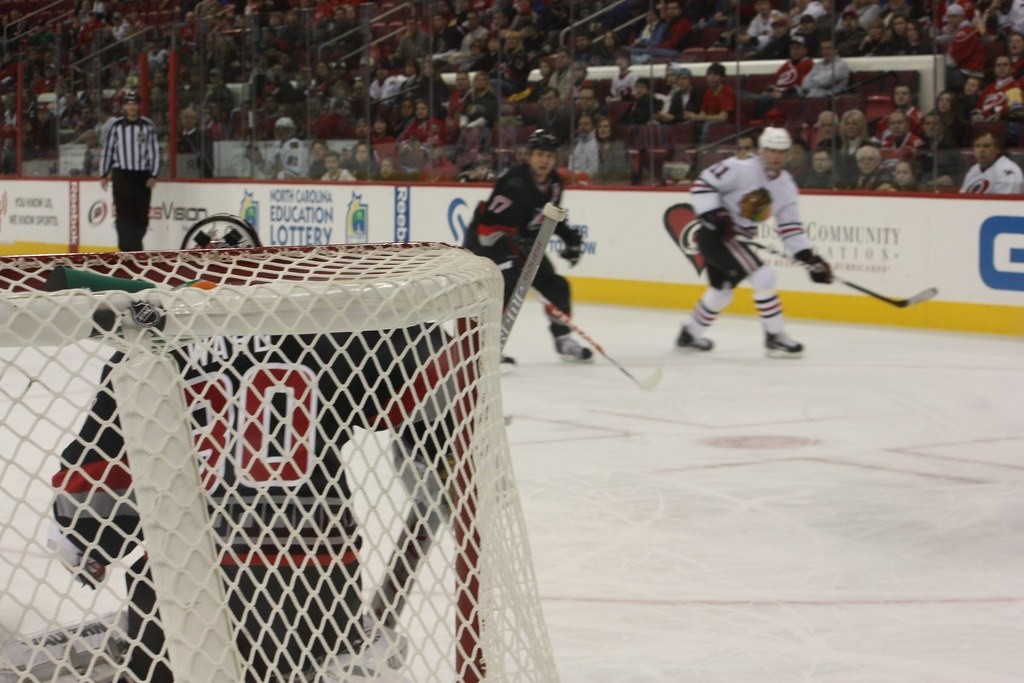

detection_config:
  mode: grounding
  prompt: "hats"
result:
[842,10,859,19]
[947,4,964,15]
[771,19,788,29]
[789,32,808,44]
[800,15,815,23]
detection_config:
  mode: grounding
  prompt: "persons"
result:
[0,0,1024,195]
[99,90,160,252]
[462,129,595,377]
[52,212,463,683]
[674,126,832,358]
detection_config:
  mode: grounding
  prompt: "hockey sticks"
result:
[735,233,938,308]
[529,285,662,390]
[365,201,566,628]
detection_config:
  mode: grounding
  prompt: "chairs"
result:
[0,0,1024,184]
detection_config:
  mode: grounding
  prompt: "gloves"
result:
[806,255,832,284]
[711,212,734,242]
[561,232,583,267]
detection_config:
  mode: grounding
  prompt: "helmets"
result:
[274,117,295,137]
[759,127,791,152]
[527,129,559,155]
[124,92,141,103]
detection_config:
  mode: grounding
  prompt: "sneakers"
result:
[555,336,594,364]
[677,329,715,352]
[764,331,804,360]
